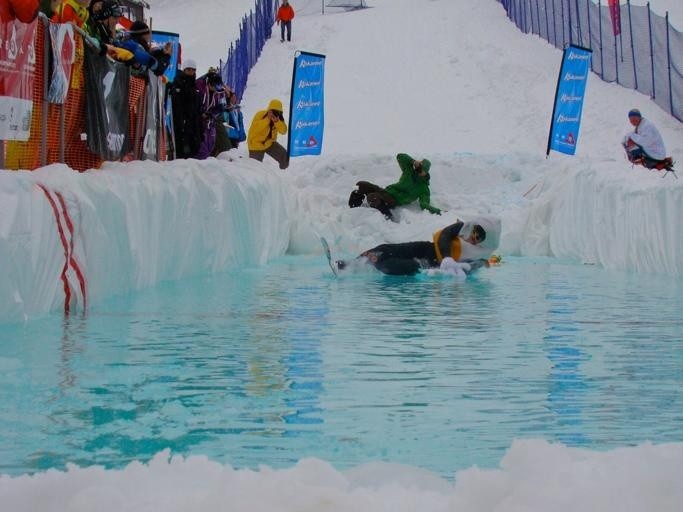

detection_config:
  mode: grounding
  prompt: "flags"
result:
[606,0,621,36]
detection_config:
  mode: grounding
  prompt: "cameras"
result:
[415,164,422,173]
[272,110,280,117]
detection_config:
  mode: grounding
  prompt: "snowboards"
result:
[321,237,341,277]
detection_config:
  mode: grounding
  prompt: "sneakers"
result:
[349,190,365,209]
[378,207,394,219]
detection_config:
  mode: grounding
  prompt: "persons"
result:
[246,99,288,169]
[333,214,502,281]
[347,152,448,220]
[621,108,675,172]
[274,0,294,43]
[0,0,246,160]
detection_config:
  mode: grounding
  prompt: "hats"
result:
[628,109,643,121]
[128,21,151,36]
[209,74,225,94]
[183,59,199,70]
[95,1,124,20]
